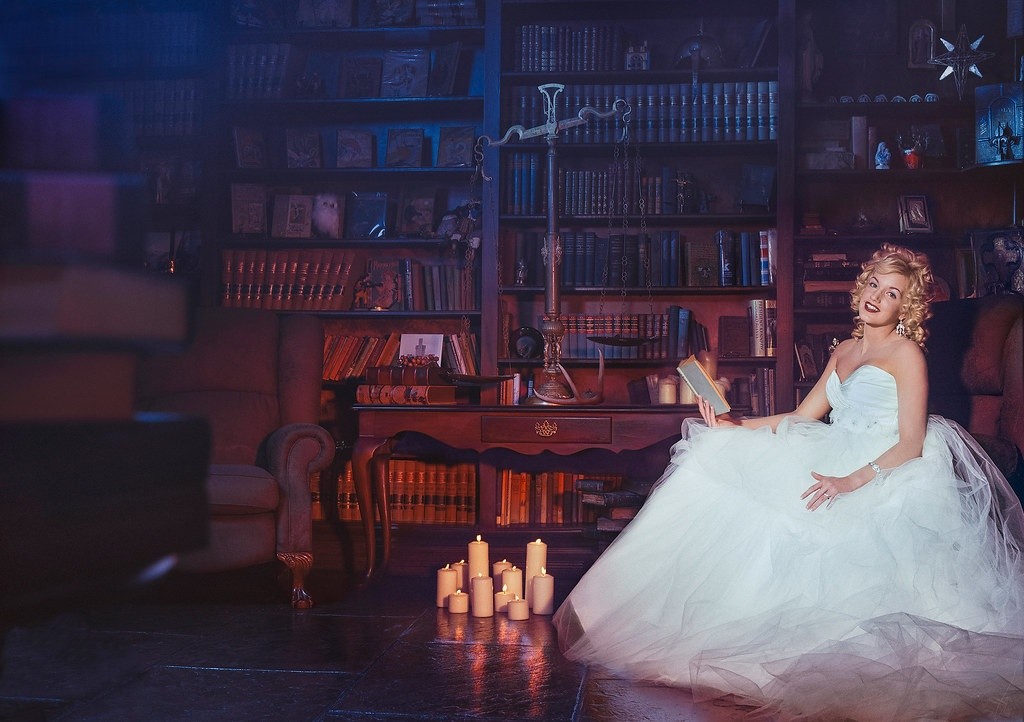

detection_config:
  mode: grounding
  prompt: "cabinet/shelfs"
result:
[58,0,958,530]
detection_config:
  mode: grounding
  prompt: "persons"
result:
[552,243,1023,722]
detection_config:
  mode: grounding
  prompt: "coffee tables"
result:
[342,402,756,580]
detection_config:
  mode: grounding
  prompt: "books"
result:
[0,3,967,530]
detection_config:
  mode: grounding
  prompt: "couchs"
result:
[140,305,337,610]
[924,296,1024,463]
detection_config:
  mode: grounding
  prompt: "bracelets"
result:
[869,462,880,475]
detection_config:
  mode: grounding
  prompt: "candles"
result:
[534,566,554,615]
[451,559,469,592]
[436,563,456,607]
[499,566,522,600]
[469,572,494,618]
[493,558,512,591]
[524,538,547,608]
[493,585,515,613]
[508,598,530,621]
[448,590,469,614]
[467,534,489,593]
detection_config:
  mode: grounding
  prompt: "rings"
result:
[824,494,828,497]
